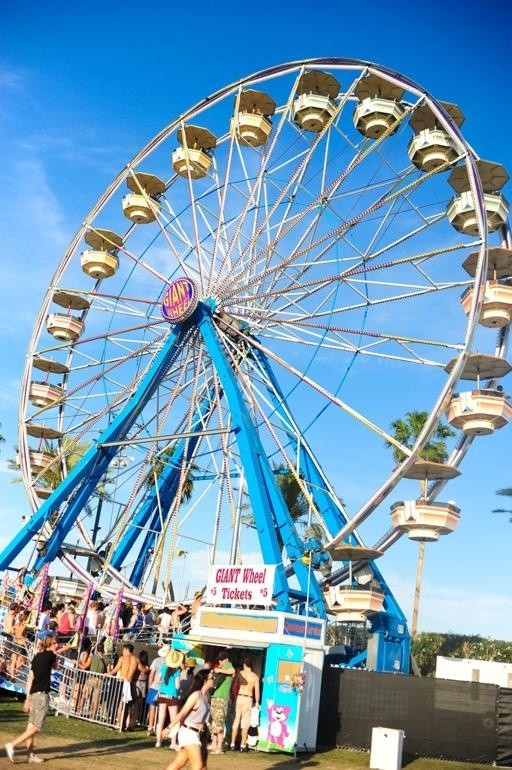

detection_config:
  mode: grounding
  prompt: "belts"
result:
[183,724,202,732]
[238,694,253,698]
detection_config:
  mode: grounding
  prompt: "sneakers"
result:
[147,728,154,736]
[208,742,248,755]
[156,742,180,751]
[5,743,14,762]
[29,756,43,763]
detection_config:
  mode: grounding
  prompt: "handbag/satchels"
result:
[200,729,211,744]
[250,707,260,728]
[154,617,161,625]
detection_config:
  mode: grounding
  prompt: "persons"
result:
[146,645,259,754]
[161,669,216,770]
[62,627,150,731]
[4,635,59,764]
[83,597,119,634]
[116,591,202,643]
[1,600,77,684]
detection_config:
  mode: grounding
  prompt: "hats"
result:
[158,644,174,657]
[91,591,103,600]
[215,650,229,660]
[166,650,184,667]
[194,591,202,599]
[185,658,198,668]
